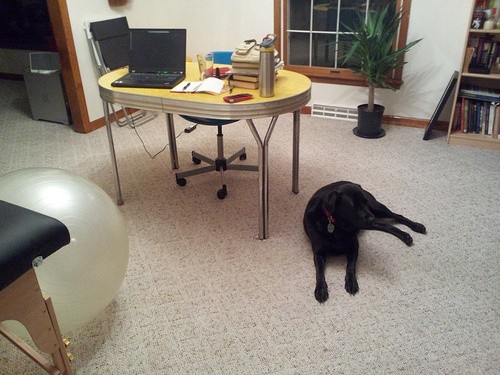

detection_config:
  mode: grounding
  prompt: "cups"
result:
[197,52,214,81]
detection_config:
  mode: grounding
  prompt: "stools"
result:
[174,114,247,199]
[0,197,75,375]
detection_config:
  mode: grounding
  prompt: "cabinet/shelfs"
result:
[24,63,72,126]
[446,0,500,150]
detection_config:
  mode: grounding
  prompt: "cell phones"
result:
[224,93,253,103]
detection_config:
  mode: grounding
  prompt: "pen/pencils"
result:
[216,67,219,78]
[229,85,234,91]
[183,82,191,90]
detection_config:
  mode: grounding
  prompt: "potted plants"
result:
[326,0,422,139]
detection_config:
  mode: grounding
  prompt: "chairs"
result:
[325,8,367,67]
[84,16,158,127]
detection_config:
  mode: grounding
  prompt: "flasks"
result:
[259,33,277,98]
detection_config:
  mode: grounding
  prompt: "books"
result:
[467,35,500,74]
[171,76,225,96]
[452,85,500,139]
[225,41,285,90]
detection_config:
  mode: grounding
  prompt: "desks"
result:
[314,4,380,63]
[98,63,312,239]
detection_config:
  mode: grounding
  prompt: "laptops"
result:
[111,29,186,89]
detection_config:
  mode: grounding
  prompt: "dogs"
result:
[303,181,426,303]
